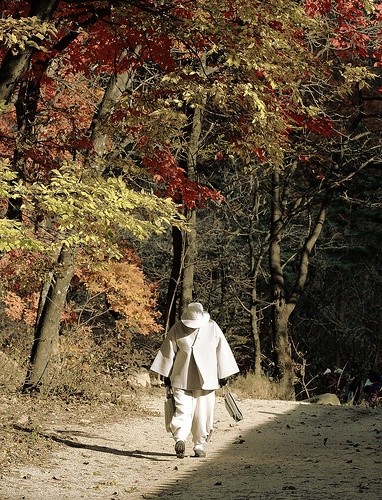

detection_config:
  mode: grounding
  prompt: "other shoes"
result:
[195,449,206,458]
[175,440,185,459]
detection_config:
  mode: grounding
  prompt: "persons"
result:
[149,302,239,458]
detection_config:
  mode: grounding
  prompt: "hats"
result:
[181,302,210,329]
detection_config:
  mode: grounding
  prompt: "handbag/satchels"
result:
[221,383,243,422]
[164,388,175,432]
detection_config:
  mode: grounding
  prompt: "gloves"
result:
[164,377,172,389]
[219,378,227,388]
